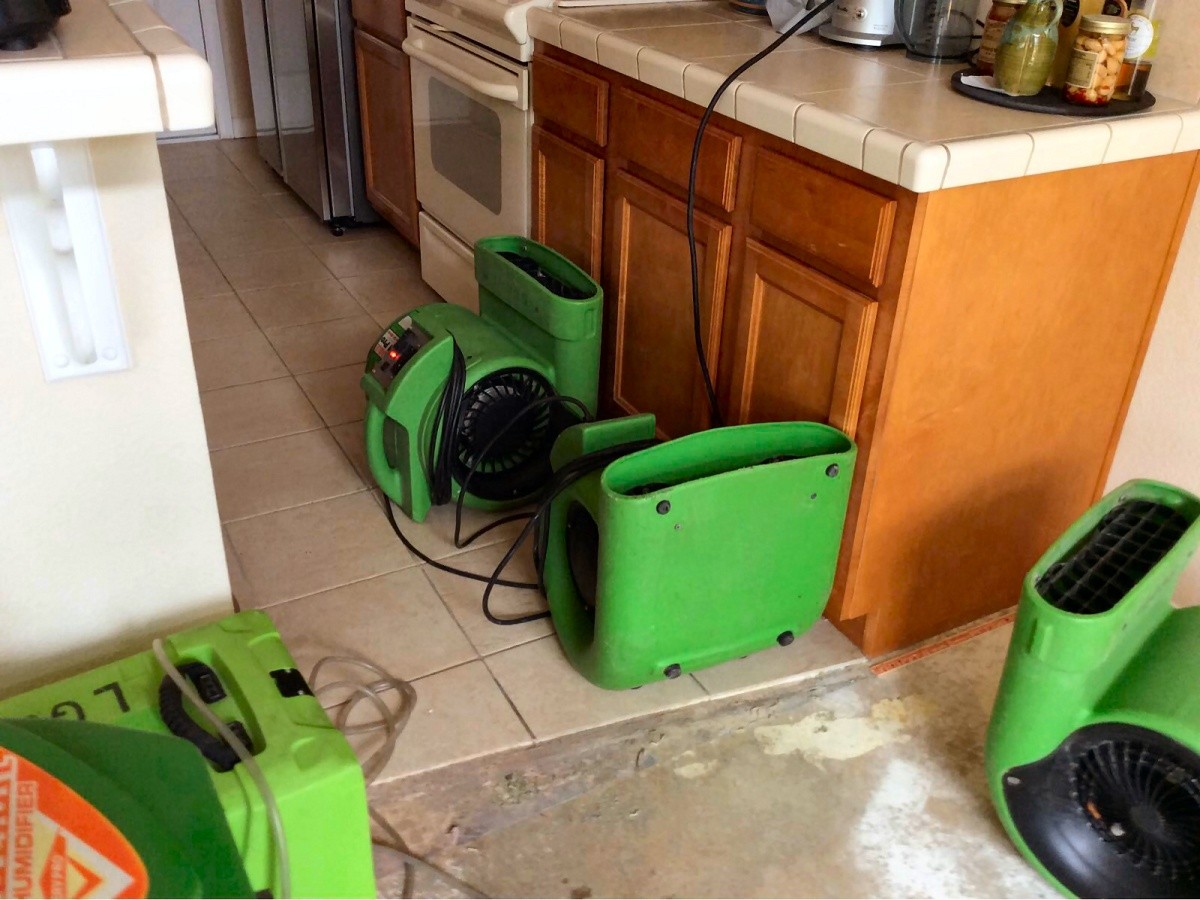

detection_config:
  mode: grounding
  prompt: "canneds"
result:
[1062,14,1132,105]
[976,0,1029,74]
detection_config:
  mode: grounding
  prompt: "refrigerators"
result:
[241,1,384,225]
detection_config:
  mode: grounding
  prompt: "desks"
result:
[0,0,239,700]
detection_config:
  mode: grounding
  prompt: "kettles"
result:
[818,0,907,47]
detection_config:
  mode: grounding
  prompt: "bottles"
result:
[1112,0,1167,102]
[1063,15,1130,108]
[977,1,1027,73]
[994,0,1063,95]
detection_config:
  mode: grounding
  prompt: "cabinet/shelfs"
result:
[352,0,416,250]
[531,40,1200,658]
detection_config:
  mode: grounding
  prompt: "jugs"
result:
[895,0,980,65]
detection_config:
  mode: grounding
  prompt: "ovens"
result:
[401,16,531,316]
